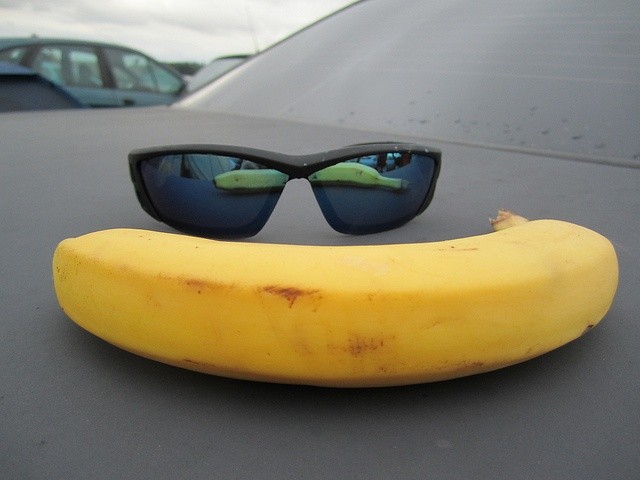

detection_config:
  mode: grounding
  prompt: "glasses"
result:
[128,142,441,240]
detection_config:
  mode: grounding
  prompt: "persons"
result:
[78,64,97,88]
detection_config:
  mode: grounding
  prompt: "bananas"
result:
[307,162,410,195]
[52,209,619,388]
[213,167,291,192]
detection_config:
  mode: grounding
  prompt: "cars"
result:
[0,34,187,108]
[0,57,86,108]
[177,54,252,103]
